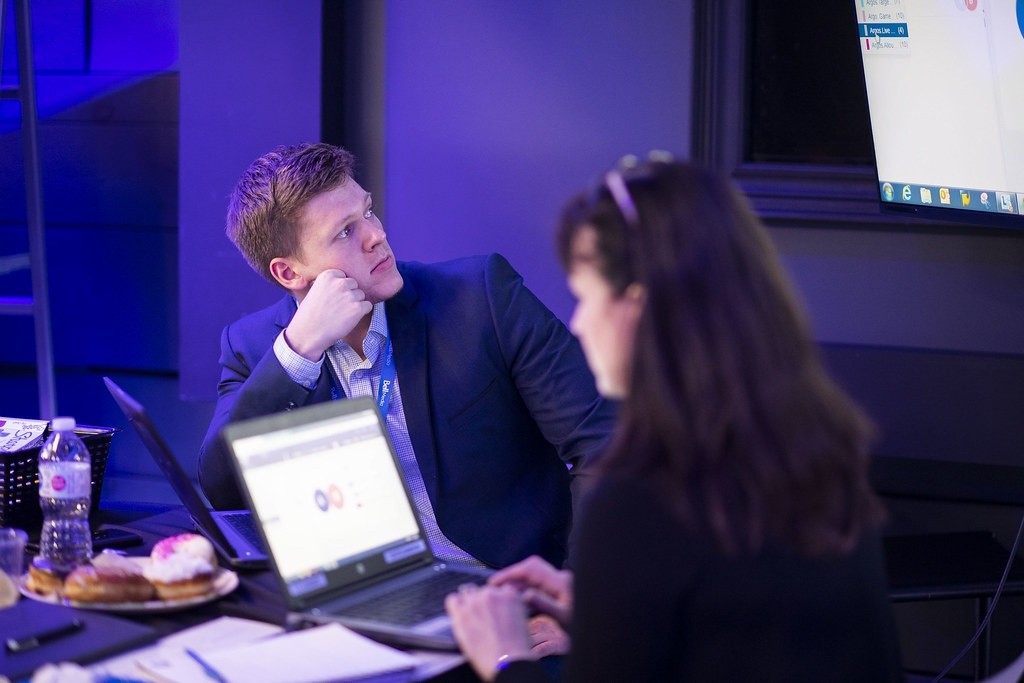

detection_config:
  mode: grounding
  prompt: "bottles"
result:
[37,416,93,572]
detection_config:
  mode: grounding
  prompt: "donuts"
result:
[28,533,217,603]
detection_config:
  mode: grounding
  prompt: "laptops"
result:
[103,377,272,570]
[218,395,541,650]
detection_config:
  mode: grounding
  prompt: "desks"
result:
[0,499,568,683]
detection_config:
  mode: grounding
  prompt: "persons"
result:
[196,142,620,660]
[444,157,888,683]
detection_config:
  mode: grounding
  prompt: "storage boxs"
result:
[1,423,113,535]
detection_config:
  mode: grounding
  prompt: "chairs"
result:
[871,457,1024,683]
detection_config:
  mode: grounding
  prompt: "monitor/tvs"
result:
[855,0,1024,224]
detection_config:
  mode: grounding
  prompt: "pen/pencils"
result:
[6,621,86,654]
[187,650,228,683]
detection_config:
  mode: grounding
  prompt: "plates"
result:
[16,554,240,612]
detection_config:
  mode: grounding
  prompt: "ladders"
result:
[0,0,55,421]
